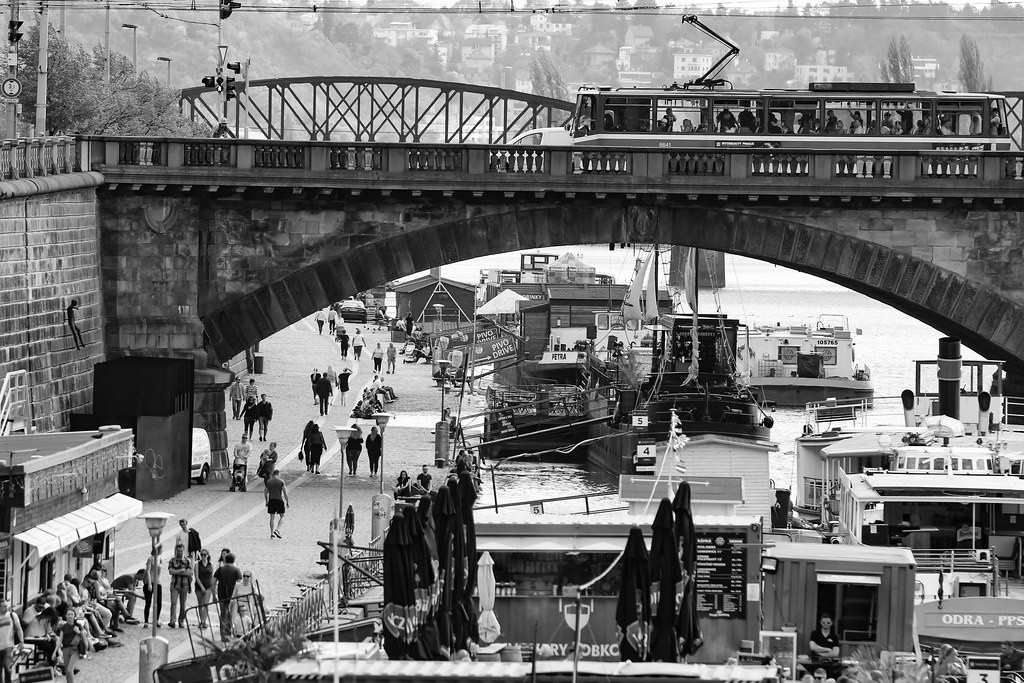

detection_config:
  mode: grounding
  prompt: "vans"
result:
[192,427,212,486]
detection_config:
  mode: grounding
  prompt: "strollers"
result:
[228,457,249,492]
[403,342,418,364]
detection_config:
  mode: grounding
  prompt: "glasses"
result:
[243,575,250,578]
[201,554,206,556]
[242,437,247,439]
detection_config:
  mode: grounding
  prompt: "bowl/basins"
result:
[781,627,796,632]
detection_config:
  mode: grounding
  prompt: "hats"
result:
[37,597,45,606]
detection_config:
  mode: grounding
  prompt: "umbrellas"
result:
[382,470,501,660]
[617,483,703,663]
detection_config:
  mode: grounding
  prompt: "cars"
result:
[489,126,577,172]
[337,299,369,324]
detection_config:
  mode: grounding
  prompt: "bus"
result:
[563,82,1024,180]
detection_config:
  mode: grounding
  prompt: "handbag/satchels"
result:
[320,432,325,448]
[336,372,338,387]
[257,468,265,478]
[298,449,303,460]
[70,634,86,659]
[10,612,20,645]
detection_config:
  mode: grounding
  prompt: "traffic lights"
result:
[227,61,240,74]
[216,75,224,94]
[226,77,236,101]
[222,0,241,19]
[8,20,24,42]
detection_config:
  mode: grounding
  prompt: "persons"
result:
[937,644,967,675]
[808,616,839,657]
[660,106,1000,136]
[0,519,270,683]
[229,294,485,540]
[564,642,585,662]
[1001,641,1024,683]
[727,655,859,683]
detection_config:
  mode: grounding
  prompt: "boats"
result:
[439,239,1024,599]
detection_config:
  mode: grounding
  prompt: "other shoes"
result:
[90,616,140,652]
[156,622,161,628]
[198,623,207,628]
[249,438,252,441]
[259,436,262,441]
[144,622,149,628]
[168,623,175,627]
[274,530,281,538]
[263,438,267,441]
[178,620,185,628]
[52,670,63,676]
[307,468,379,478]
[271,535,275,539]
[373,370,398,403]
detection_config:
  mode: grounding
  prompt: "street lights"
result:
[137,510,175,638]
[122,23,138,72]
[436,359,452,422]
[157,56,172,87]
[371,409,393,495]
[334,426,357,518]
[432,303,445,335]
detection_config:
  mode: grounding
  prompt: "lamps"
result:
[759,555,779,574]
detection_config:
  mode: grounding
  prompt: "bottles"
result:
[473,584,478,595]
[495,552,613,596]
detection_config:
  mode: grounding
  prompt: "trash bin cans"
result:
[254,356,263,373]
[740,639,754,653]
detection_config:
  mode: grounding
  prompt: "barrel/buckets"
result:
[477,646,523,662]
